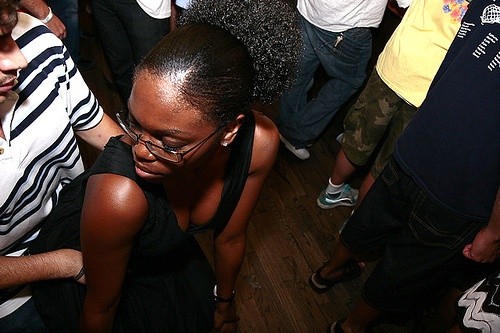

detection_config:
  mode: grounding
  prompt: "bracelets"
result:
[74,267,85,280]
[213,285,235,304]
[39,6,54,23]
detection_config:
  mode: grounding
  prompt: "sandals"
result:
[330,320,356,333]
[309,260,365,292]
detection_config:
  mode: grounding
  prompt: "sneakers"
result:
[316,183,360,208]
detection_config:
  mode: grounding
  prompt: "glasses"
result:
[115,108,232,164]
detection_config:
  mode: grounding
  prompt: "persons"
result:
[27,0,305,333]
[309,1,500,333]
[419,260,499,333]
[91,0,178,119]
[1,0,28,105]
[1,9,132,331]
[316,1,470,236]
[16,0,88,87]
[276,0,390,159]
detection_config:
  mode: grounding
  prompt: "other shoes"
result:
[279,133,310,159]
